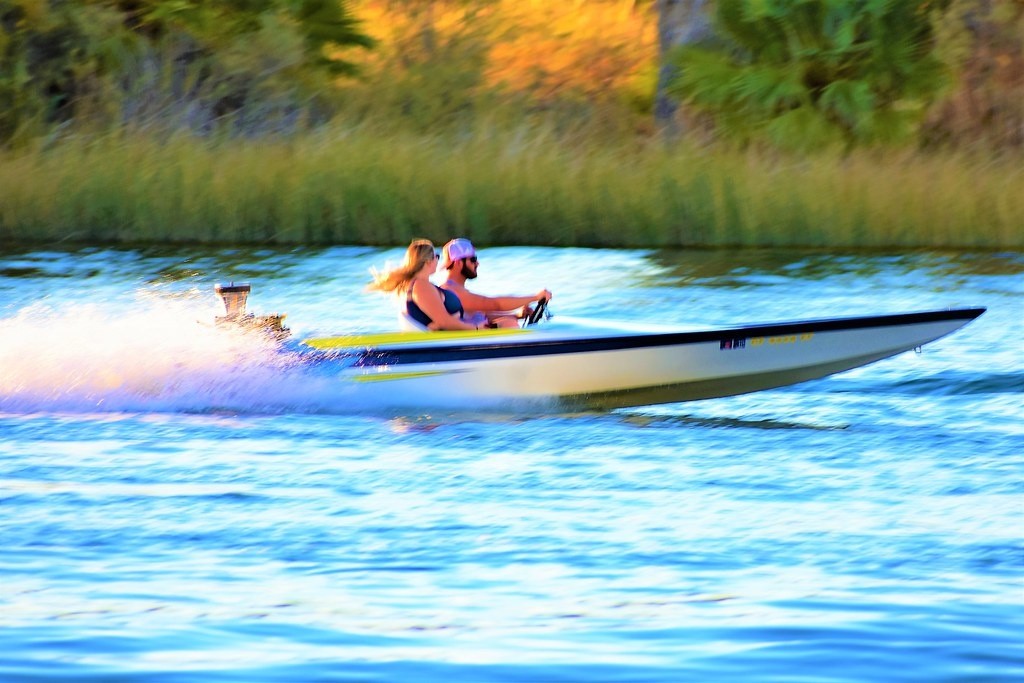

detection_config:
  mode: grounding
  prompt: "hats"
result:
[436,238,476,270]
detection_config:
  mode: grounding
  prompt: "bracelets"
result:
[477,325,478,330]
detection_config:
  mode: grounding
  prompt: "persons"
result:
[439,238,552,318]
[387,242,519,332]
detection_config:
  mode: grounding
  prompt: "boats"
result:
[211,278,986,416]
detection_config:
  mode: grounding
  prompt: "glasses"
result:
[436,255,440,260]
[470,256,478,262]
